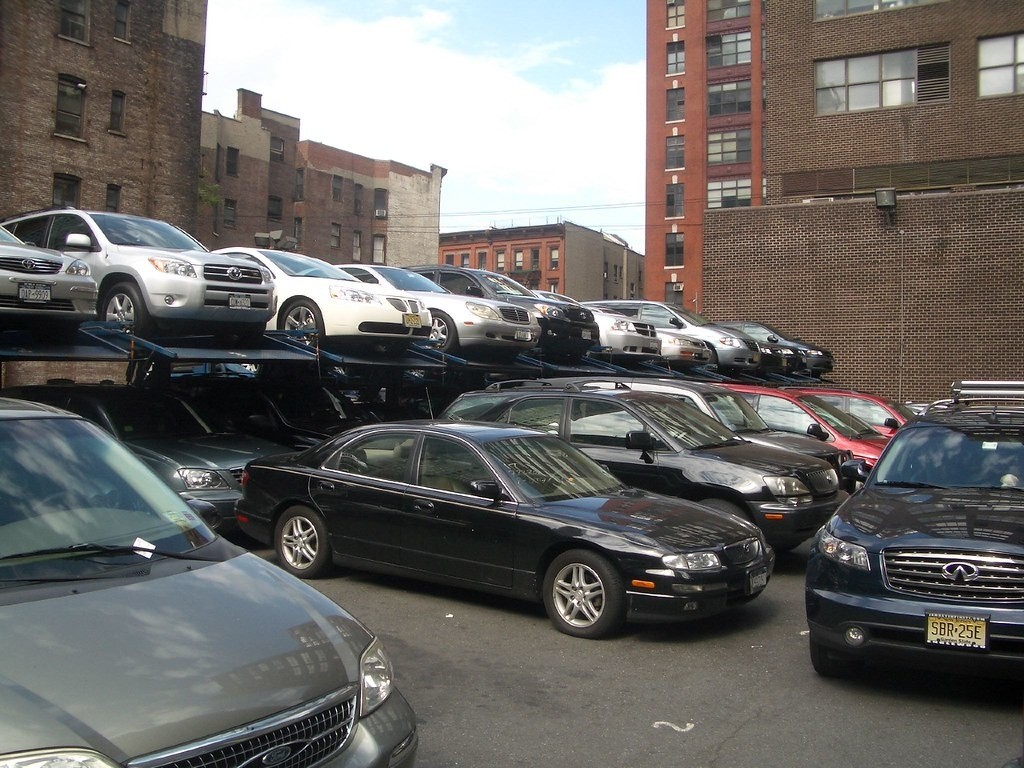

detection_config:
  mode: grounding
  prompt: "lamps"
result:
[875,188,897,225]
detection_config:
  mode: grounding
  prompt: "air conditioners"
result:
[674,284,684,291]
[376,210,386,218]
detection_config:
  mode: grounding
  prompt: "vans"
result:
[579,301,762,377]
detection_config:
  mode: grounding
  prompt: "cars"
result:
[175,373,392,456]
[2,381,297,533]
[348,372,448,419]
[331,264,542,359]
[234,419,772,637]
[210,247,433,350]
[497,290,662,366]
[0,226,98,339]
[756,342,808,375]
[587,305,712,369]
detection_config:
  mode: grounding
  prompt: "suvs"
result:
[712,322,834,378]
[804,378,1024,676]
[402,266,600,361]
[488,376,843,486]
[777,387,915,437]
[1,396,420,767]
[711,382,892,470]
[0,207,277,349]
[472,382,850,548]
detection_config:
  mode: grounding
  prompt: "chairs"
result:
[396,442,487,494]
